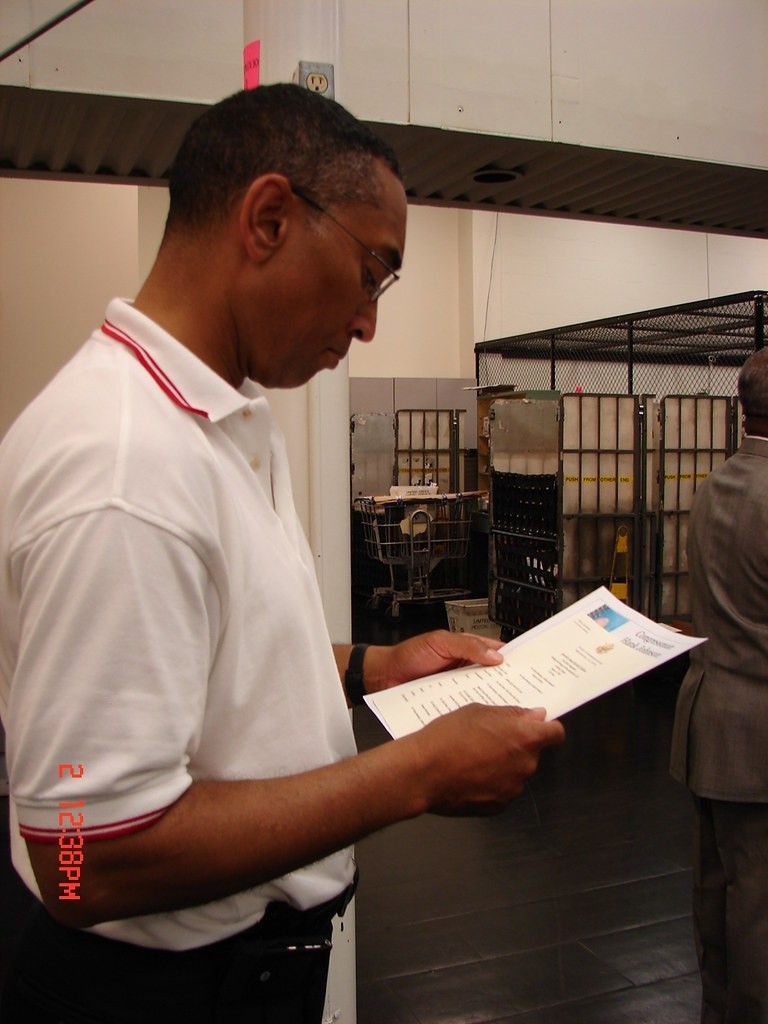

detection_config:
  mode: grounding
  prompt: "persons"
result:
[1,82,565,1024]
[669,346,768,1024]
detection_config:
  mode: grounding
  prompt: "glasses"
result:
[290,186,400,302]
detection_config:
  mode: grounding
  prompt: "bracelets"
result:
[346,644,370,708]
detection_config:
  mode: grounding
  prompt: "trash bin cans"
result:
[445,598,502,644]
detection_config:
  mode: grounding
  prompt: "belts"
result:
[64,866,358,960]
